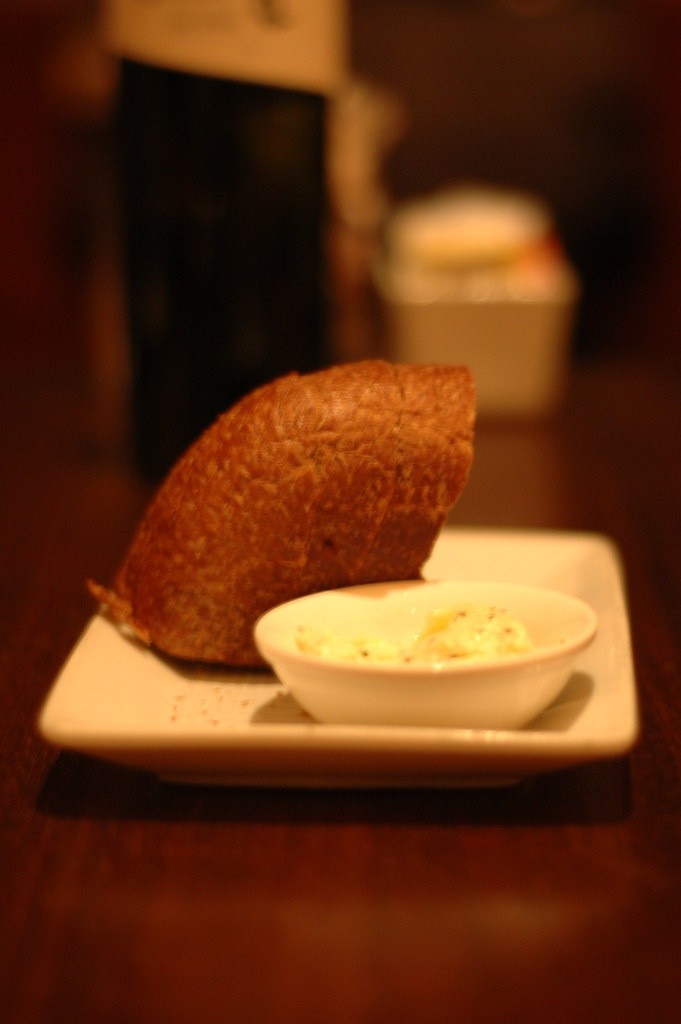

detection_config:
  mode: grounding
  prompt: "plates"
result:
[35,525,641,788]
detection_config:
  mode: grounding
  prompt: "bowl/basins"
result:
[252,580,598,729]
[370,262,581,422]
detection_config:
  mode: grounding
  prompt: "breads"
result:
[83,364,477,663]
